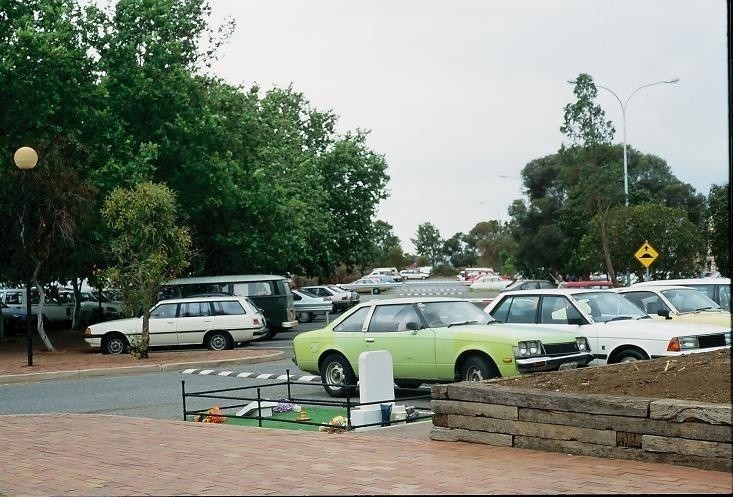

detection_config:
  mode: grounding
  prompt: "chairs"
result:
[647,302,665,314]
[493,308,537,322]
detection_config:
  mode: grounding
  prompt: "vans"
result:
[153,272,298,339]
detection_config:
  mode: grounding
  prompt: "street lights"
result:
[567,78,678,207]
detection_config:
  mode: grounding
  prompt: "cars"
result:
[293,294,594,396]
[455,265,515,292]
[337,264,405,295]
[292,274,360,323]
[485,276,731,366]
[401,268,429,280]
[84,291,269,353]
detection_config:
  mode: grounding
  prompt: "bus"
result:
[5,289,124,329]
